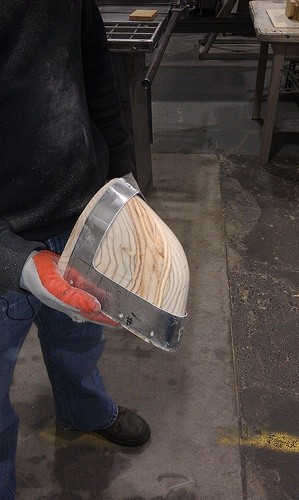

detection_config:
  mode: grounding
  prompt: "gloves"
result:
[19,250,121,327]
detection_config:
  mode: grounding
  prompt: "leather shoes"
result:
[96,406,151,445]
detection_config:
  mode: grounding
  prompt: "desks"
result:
[249,0,299,165]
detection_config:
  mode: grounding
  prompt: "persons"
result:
[1,1,150,500]
